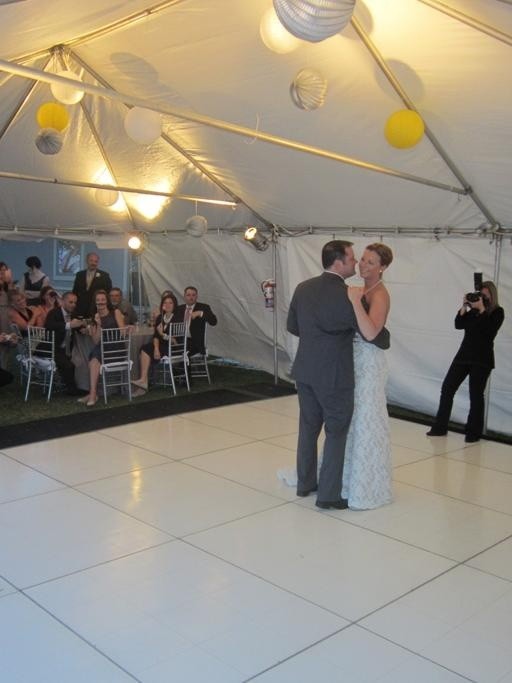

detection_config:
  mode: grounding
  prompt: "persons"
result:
[152,290,172,319]
[130,294,178,397]
[0,263,12,292]
[107,287,138,324]
[33,291,89,396]
[31,286,63,327]
[73,253,112,312]
[18,256,49,305]
[7,289,45,338]
[172,286,217,388]
[77,290,126,406]
[426,281,504,442]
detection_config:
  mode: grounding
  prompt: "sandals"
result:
[87,395,99,406]
[77,395,89,402]
[131,389,146,397]
[130,379,148,390]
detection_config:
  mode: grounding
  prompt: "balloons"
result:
[123,106,162,145]
[51,70,85,105]
[260,5,302,55]
[36,101,71,133]
[383,108,425,149]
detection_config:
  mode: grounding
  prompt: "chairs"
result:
[101,327,133,403]
[24,326,56,403]
[158,322,190,396]
[189,321,211,383]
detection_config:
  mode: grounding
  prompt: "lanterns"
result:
[95,188,118,206]
[273,0,355,43]
[290,69,326,112]
[185,215,208,238]
[35,128,63,155]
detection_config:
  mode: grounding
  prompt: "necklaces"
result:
[364,280,382,296]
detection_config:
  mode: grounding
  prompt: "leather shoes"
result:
[296,485,318,497]
[315,499,348,510]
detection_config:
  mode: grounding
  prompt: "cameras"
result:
[466,272,489,309]
[49,291,57,297]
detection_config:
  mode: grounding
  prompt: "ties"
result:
[86,271,94,291]
[184,305,192,322]
[65,314,72,357]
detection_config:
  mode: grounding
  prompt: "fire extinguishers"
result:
[261,279,276,307]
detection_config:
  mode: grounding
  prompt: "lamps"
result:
[245,229,270,251]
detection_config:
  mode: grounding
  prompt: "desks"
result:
[129,324,155,389]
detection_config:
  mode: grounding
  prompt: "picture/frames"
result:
[53,240,84,281]
